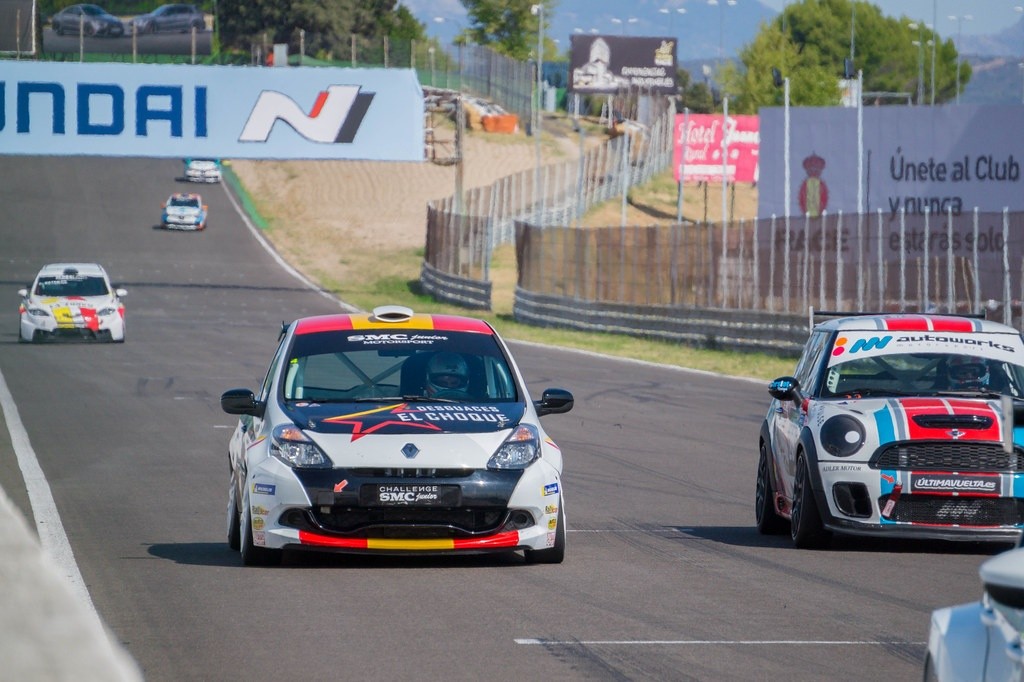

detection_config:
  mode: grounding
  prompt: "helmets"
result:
[945,354,991,389]
[424,353,471,401]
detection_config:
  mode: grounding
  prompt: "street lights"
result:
[843,58,864,312]
[947,17,974,104]
[708,1,738,63]
[611,18,639,34]
[908,24,933,106]
[530,3,544,132]
[773,69,789,312]
[660,9,686,35]
[434,18,468,79]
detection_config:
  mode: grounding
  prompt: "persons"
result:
[424,352,470,399]
[946,355,990,392]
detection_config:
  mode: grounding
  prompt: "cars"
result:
[49,4,123,38]
[756,304,1024,546]
[923,546,1022,682]
[161,194,208,231]
[17,264,128,345]
[220,306,575,564]
[130,3,207,35]
[185,158,225,184]
